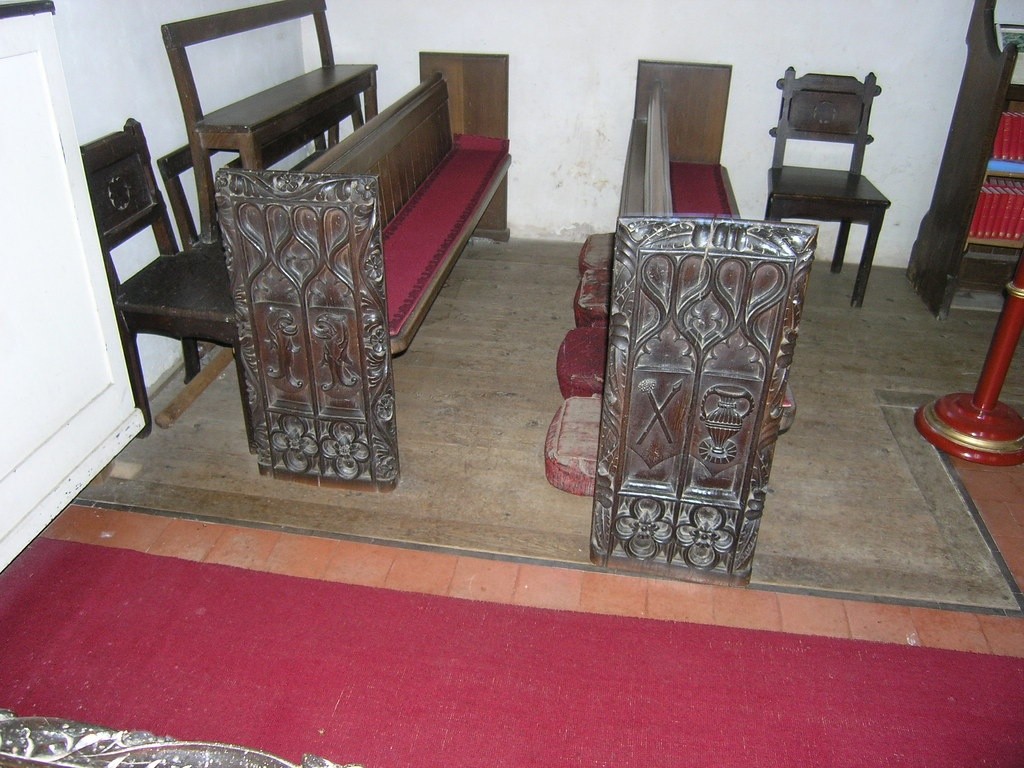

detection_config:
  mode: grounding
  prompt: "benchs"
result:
[156,0,821,589]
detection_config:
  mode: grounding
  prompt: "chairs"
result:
[76,119,258,455]
[766,65,890,307]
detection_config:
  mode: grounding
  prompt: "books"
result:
[989,110,1024,162]
[967,176,1024,241]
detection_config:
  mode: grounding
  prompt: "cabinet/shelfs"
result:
[904,0,1023,321]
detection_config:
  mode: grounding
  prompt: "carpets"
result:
[0,535,1024,768]
